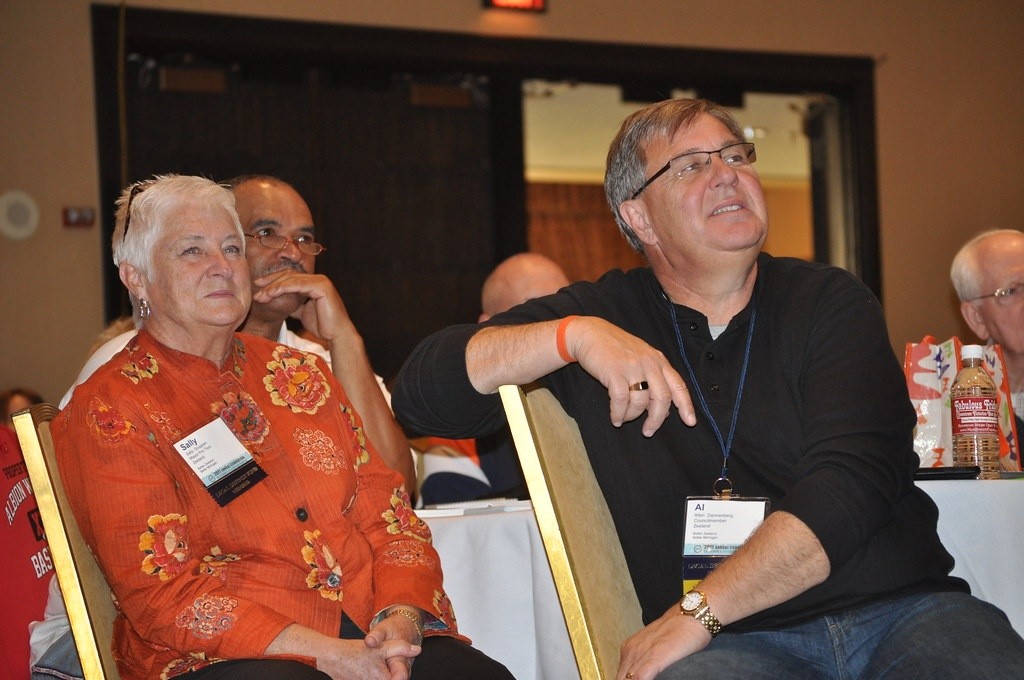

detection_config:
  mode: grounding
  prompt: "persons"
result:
[398,96,1024,680]
[945,229,1024,472]
[0,173,568,680]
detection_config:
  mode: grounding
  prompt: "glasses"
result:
[123,182,162,244]
[964,283,1024,305]
[632,143,756,200]
[244,233,326,255]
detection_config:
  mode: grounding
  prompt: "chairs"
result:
[13,403,122,680]
[1,423,57,679]
[498,384,647,680]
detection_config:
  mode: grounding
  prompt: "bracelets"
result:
[556,316,579,363]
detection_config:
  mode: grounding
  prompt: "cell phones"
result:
[914,467,982,481]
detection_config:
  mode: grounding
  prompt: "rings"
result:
[629,381,649,390]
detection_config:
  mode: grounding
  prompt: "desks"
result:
[419,479,1024,680]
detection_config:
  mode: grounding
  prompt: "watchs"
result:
[678,590,724,639]
[384,608,425,646]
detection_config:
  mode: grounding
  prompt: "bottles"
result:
[949,344,999,479]
